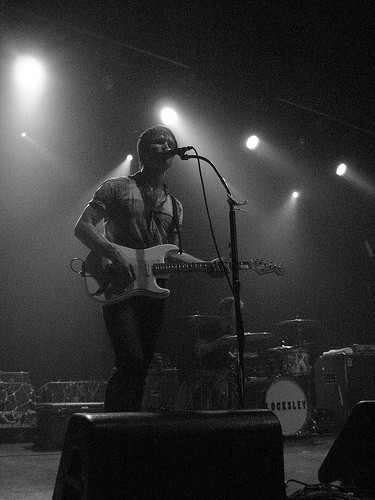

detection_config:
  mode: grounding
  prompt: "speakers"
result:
[316,400,375,500]
[53,408,287,500]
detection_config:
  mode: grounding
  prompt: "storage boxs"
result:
[35,402,104,446]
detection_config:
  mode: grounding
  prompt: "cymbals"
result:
[217,332,275,346]
[175,315,222,321]
[264,345,294,351]
[275,319,318,327]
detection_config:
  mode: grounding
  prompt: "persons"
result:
[196,296,268,410]
[72,125,233,413]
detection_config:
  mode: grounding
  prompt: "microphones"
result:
[168,145,193,157]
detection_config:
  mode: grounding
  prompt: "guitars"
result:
[83,243,285,305]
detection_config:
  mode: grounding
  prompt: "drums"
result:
[243,374,312,437]
[280,348,311,377]
[224,330,260,360]
[192,369,226,410]
